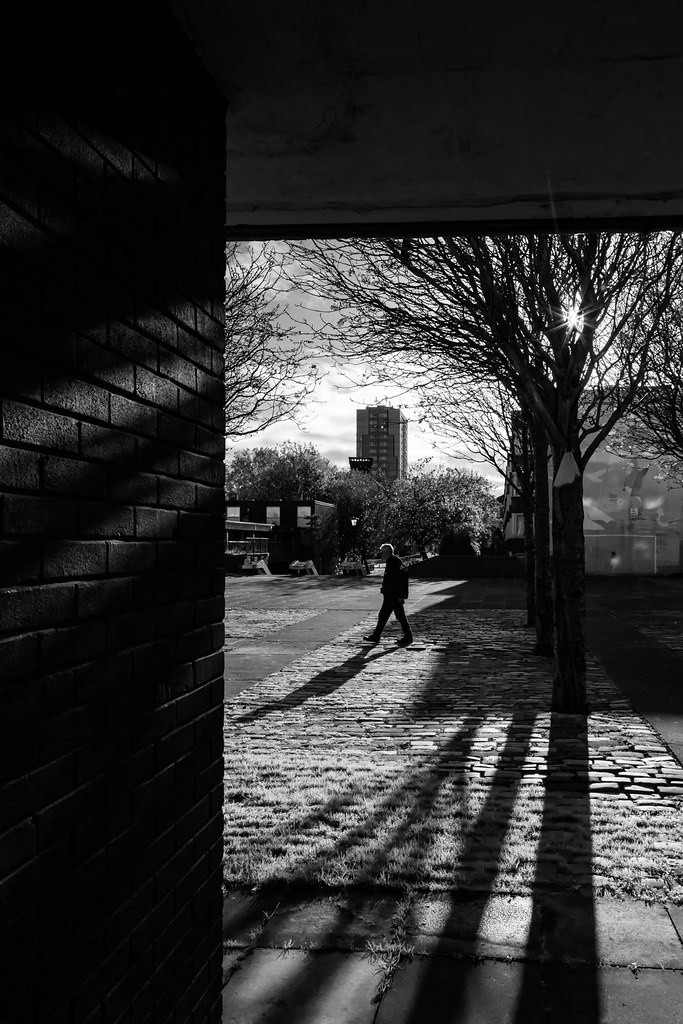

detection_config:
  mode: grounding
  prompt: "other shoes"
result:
[398,635,413,643]
[364,635,380,643]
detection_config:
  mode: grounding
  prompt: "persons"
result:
[363,543,414,647]
